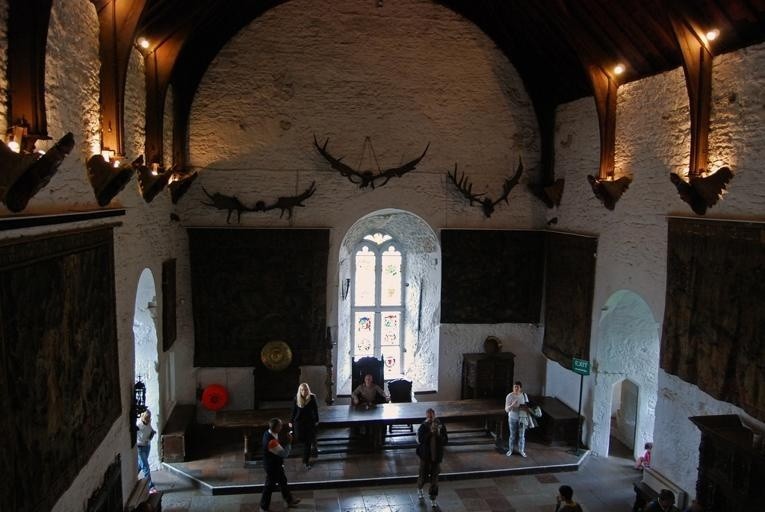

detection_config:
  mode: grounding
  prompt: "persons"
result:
[414,407,449,510]
[257,416,302,512]
[554,484,584,511]
[136,409,158,495]
[291,382,320,470]
[351,372,389,405]
[504,381,531,457]
[632,442,653,469]
[644,488,680,511]
[684,494,709,512]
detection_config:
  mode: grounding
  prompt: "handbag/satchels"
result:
[527,413,534,429]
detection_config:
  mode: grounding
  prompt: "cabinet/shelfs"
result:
[687,414,764,512]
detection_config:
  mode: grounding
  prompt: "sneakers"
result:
[519,452,527,458]
[506,450,513,457]
[417,488,424,499]
[431,500,437,508]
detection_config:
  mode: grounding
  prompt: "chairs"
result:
[350,355,389,406]
[388,380,415,434]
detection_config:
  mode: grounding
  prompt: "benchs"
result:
[631,468,685,512]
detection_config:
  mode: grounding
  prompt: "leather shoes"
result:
[288,499,300,507]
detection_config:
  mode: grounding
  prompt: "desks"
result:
[212,397,509,470]
